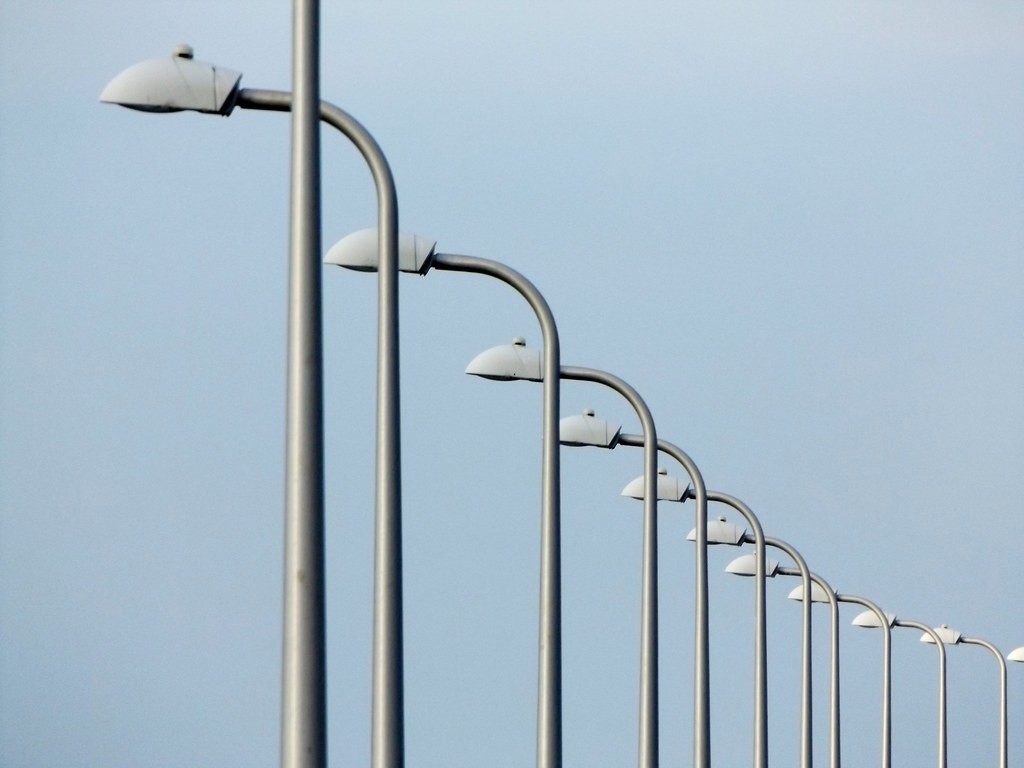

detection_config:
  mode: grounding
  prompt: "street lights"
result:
[464,335,1024,768]
[100,42,406,768]
[323,225,563,768]
